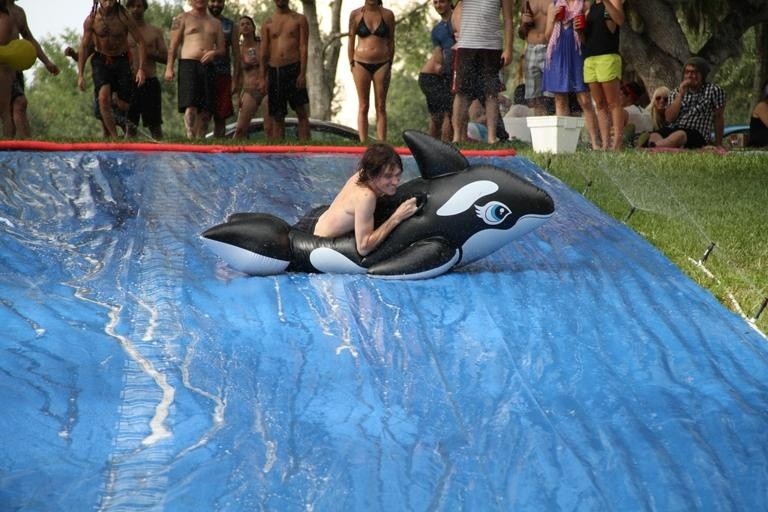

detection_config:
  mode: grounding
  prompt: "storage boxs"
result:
[525,113,587,154]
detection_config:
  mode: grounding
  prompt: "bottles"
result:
[452,69,457,92]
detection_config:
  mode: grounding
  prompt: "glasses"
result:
[684,68,700,76]
[655,95,668,103]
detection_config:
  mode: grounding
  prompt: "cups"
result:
[575,15,586,31]
[559,6,565,20]
[730,133,744,148]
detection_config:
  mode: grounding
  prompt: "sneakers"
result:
[621,123,635,146]
[636,132,654,148]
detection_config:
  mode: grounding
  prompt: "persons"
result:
[290,143,419,256]
[348,0,395,142]
